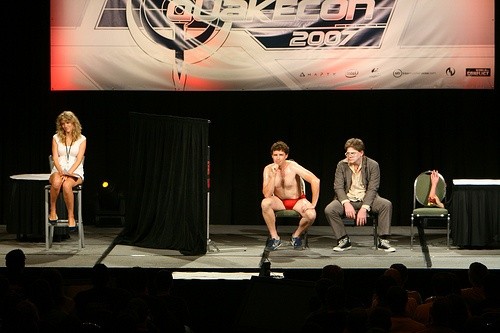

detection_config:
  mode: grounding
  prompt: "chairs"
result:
[411,170,451,247]
[273,176,308,247]
[337,211,378,250]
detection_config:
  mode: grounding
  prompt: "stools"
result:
[44,154,85,251]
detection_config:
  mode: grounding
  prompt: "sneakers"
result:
[333,237,352,252]
[264,238,282,250]
[290,236,304,248]
[378,239,395,252]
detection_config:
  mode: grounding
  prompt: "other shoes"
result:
[49,214,57,225]
[68,221,75,231]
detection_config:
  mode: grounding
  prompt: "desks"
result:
[451,179,500,250]
[9,174,71,243]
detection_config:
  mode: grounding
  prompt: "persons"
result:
[260,141,321,251]
[0,249,500,333]
[426,169,445,208]
[324,138,398,253]
[48,111,87,231]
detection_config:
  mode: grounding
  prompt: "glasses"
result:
[345,152,360,156]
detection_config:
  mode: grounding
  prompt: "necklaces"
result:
[64,133,74,162]
